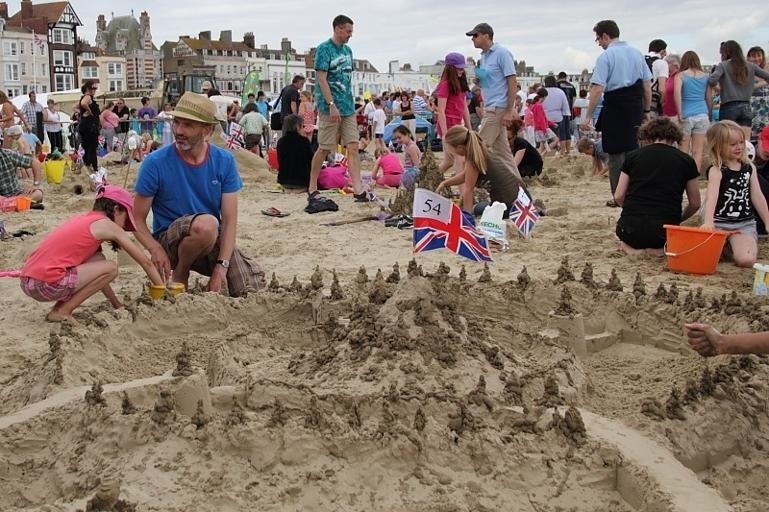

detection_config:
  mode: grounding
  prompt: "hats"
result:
[760,126,769,152]
[201,81,211,90]
[467,90,476,99]
[165,91,219,126]
[445,53,468,69]
[95,185,137,232]
[466,23,493,37]
[6,125,22,135]
[745,140,756,162]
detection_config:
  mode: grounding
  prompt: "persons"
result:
[464,24,526,186]
[580,17,655,206]
[698,120,769,269]
[132,91,267,300]
[506,72,610,180]
[684,322,768,356]
[645,39,769,233]
[0,80,173,209]
[356,52,493,212]
[18,184,171,324]
[200,76,335,190]
[614,115,702,259]
[304,13,381,205]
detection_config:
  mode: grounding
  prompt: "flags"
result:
[412,185,495,266]
[507,184,538,238]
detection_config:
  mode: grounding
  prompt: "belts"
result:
[752,91,768,95]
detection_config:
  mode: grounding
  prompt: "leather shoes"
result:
[607,198,620,207]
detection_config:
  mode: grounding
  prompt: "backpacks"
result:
[644,55,660,110]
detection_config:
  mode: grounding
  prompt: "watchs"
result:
[215,257,231,269]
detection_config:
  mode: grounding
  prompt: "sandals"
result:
[306,190,326,203]
[354,192,384,202]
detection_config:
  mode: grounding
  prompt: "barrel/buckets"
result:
[662,221,732,274]
[146,279,186,300]
[43,160,67,185]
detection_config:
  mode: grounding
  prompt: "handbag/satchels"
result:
[271,112,282,131]
[114,124,122,133]
[319,167,346,190]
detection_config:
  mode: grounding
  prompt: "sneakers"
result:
[30,201,44,211]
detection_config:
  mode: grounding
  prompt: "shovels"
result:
[319,213,393,227]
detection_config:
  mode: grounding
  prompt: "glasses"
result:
[91,87,97,90]
[508,136,514,140]
[117,102,122,105]
[472,33,485,38]
[595,32,608,42]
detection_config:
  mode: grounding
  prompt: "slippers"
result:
[261,207,290,217]
